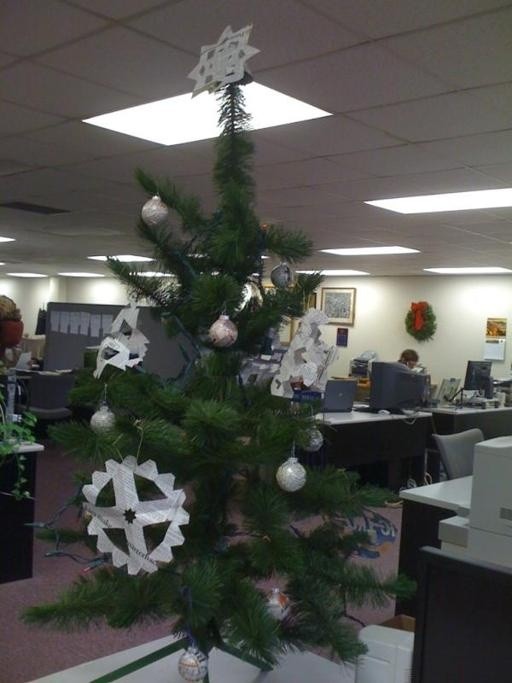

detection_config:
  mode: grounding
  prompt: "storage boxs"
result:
[354,614,416,683]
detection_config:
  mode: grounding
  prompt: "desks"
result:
[0,436,45,585]
[394,476,471,618]
[295,403,433,494]
[421,405,512,439]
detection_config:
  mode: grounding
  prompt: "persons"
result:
[399,350,418,370]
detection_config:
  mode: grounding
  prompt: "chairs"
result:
[432,428,484,479]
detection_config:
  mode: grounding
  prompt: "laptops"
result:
[322,379,358,413]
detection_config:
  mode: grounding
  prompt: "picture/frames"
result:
[319,287,356,326]
[263,285,294,346]
[304,293,316,312]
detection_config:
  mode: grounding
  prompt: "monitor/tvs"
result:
[368,361,430,415]
[465,360,494,398]
